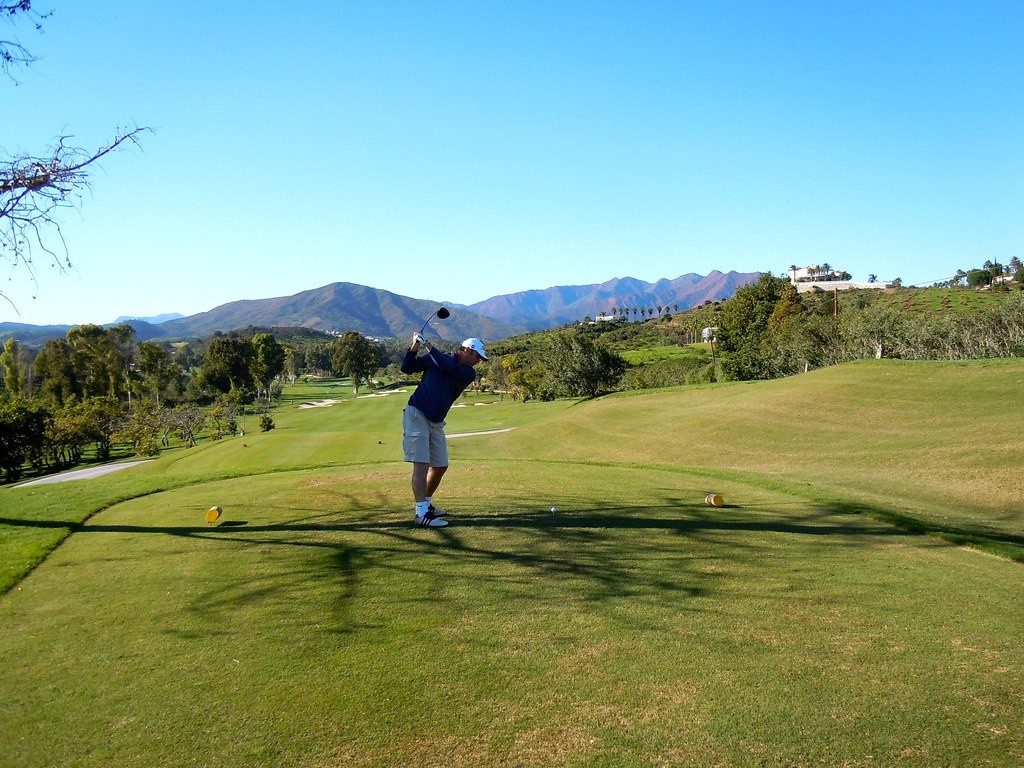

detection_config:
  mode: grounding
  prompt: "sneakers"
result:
[428,503,447,517]
[415,514,449,527]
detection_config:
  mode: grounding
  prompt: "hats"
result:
[462,338,489,362]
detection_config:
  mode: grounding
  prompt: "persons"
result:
[402,332,489,528]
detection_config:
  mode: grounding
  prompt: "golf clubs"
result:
[416,307,451,343]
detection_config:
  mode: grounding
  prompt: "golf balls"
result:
[550,507,556,512]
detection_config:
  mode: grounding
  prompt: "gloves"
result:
[414,333,428,345]
